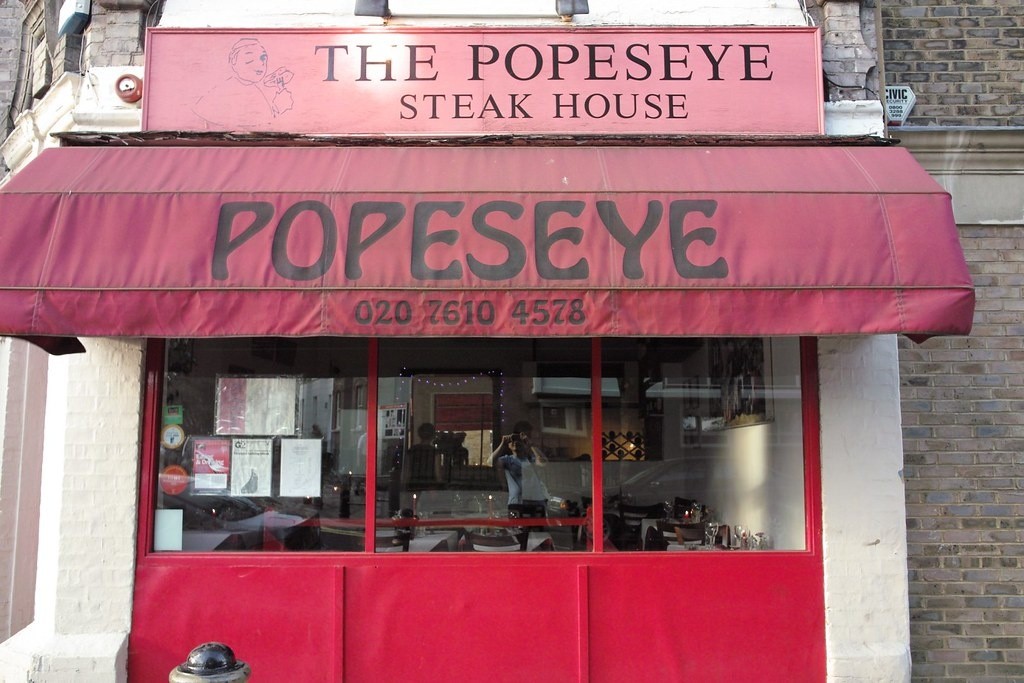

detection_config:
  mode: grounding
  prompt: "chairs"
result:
[464,530,529,552]
[350,532,410,552]
[618,497,730,547]
[507,504,546,532]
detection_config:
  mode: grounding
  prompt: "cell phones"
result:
[511,435,520,440]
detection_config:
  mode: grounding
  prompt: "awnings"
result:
[1,146,975,354]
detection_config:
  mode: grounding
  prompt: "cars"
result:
[153,474,322,553]
[546,453,732,549]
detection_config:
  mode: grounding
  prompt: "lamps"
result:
[555,0,590,22]
[354,0,392,19]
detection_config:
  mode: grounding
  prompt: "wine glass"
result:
[704,523,719,551]
[691,503,702,523]
[735,526,749,551]
[663,500,673,524]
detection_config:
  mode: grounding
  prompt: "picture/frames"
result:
[683,416,700,445]
[719,337,776,429]
[682,375,701,410]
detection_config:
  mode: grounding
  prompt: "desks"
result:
[642,518,723,551]
[369,530,458,552]
[476,532,550,552]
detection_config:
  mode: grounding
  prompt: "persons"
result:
[485,432,551,537]
[354,431,368,476]
[496,421,555,492]
[408,423,447,485]
